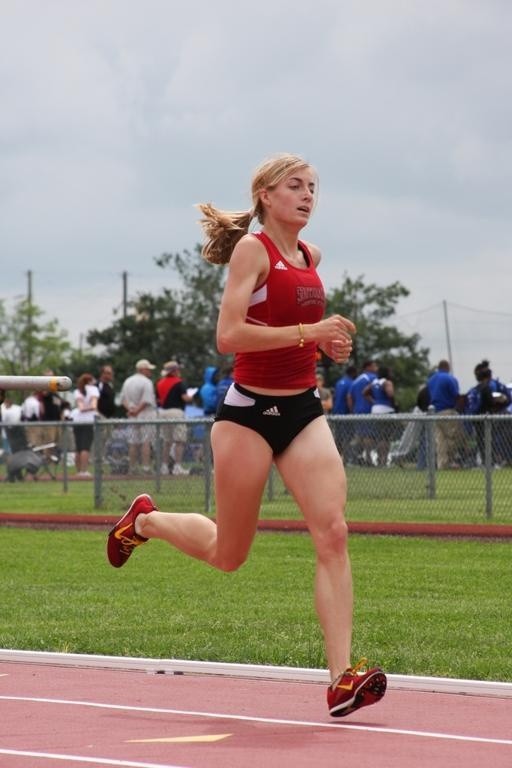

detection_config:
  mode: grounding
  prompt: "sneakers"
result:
[172,467,190,474]
[107,494,158,568]
[327,657,387,717]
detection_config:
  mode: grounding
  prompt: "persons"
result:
[316,360,396,466]
[461,361,511,466]
[105,156,388,717]
[397,360,465,469]
[185,367,234,466]
[121,361,193,475]
[1,366,114,477]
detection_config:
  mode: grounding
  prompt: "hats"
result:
[134,359,156,370]
[160,361,179,376]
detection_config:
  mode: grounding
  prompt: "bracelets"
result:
[298,323,304,348]
